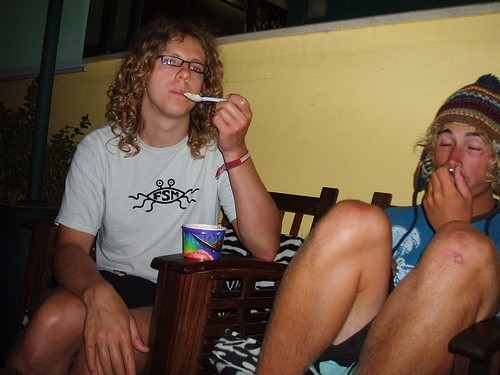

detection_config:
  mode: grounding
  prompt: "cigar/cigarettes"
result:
[449,165,455,171]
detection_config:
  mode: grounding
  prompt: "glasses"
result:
[156,55,210,74]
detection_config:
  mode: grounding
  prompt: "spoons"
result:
[183,92,226,103]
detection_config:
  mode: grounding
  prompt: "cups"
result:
[181,223,227,262]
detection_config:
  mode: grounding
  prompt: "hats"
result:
[392,73,500,279]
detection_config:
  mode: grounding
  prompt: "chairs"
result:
[156,192,500,375]
[11,185,340,375]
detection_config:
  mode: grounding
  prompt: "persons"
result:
[0,17,282,375]
[254,74,500,375]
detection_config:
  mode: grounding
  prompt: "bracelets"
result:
[216,151,250,179]
